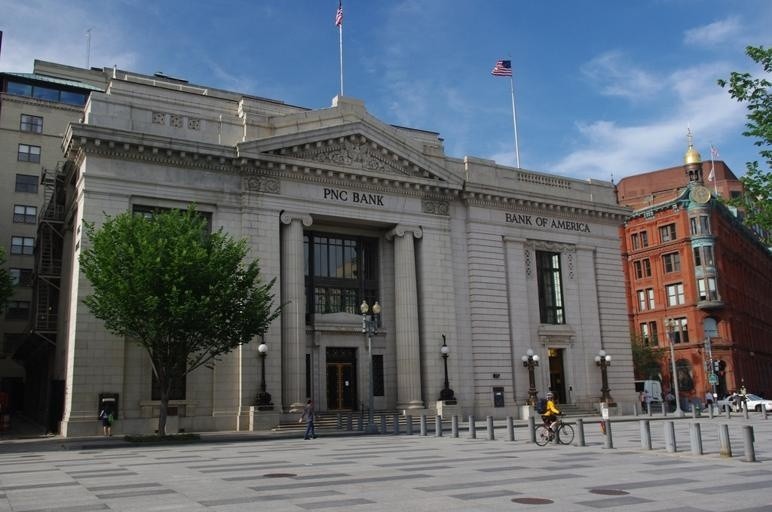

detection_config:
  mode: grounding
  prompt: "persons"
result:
[643,389,650,413]
[540,391,565,443]
[732,391,738,411]
[664,391,676,411]
[297,399,321,440]
[705,391,714,408]
[739,385,746,395]
[639,390,645,407]
[98,404,114,436]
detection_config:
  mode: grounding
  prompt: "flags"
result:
[490,60,512,77]
[335,1,343,29]
[711,145,720,158]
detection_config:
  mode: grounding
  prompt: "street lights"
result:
[361,301,381,423]
[595,350,614,406]
[522,350,540,408]
[664,316,684,416]
[257,346,272,406]
[439,333,454,401]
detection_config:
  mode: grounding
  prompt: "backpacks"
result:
[536,398,549,414]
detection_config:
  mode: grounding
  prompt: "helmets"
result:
[546,392,553,397]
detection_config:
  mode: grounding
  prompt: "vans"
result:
[635,380,663,404]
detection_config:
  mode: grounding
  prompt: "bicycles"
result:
[534,410,575,446]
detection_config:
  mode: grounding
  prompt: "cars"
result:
[716,392,772,413]
